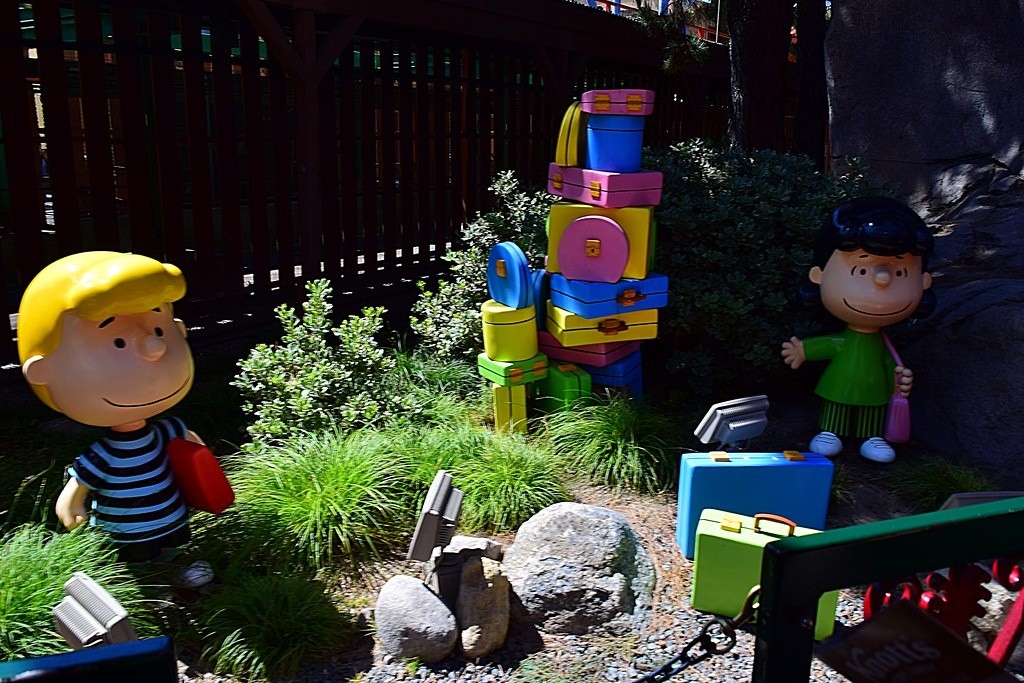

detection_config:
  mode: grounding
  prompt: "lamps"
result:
[52,571,137,649]
[694,394,771,453]
[406,468,464,563]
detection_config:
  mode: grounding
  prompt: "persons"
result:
[781,193,936,467]
[17,249,215,612]
